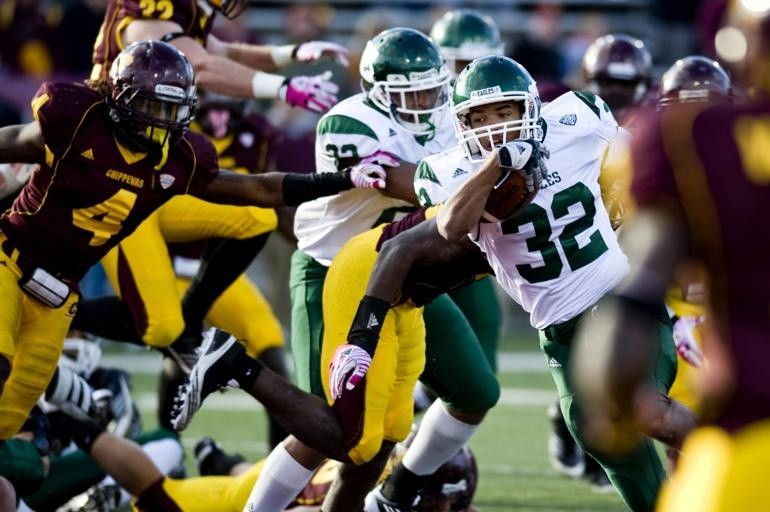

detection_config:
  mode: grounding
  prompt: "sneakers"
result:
[170,327,248,430]
[194,436,217,476]
[363,483,401,512]
[550,406,585,476]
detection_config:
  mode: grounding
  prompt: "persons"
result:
[0,1,770,512]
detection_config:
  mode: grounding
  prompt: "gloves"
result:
[329,344,370,400]
[278,42,348,114]
[349,150,401,189]
[497,139,550,192]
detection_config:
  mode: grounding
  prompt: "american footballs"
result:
[480,165,539,223]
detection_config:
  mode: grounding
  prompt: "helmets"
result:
[582,36,730,108]
[422,445,477,512]
[359,9,543,164]
[106,40,197,151]
